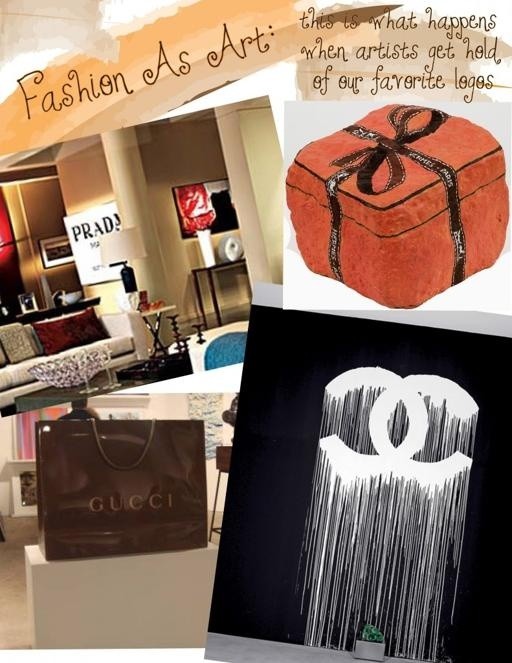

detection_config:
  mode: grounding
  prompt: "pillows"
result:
[0,308,117,368]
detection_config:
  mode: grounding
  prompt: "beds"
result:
[15,367,185,412]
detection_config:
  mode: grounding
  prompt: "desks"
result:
[128,304,179,378]
[189,255,254,328]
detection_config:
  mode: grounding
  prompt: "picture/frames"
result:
[172,177,241,238]
[17,290,40,312]
[37,233,77,270]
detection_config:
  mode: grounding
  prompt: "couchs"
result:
[0,307,148,412]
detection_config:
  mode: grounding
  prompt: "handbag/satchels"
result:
[34,421,208,561]
